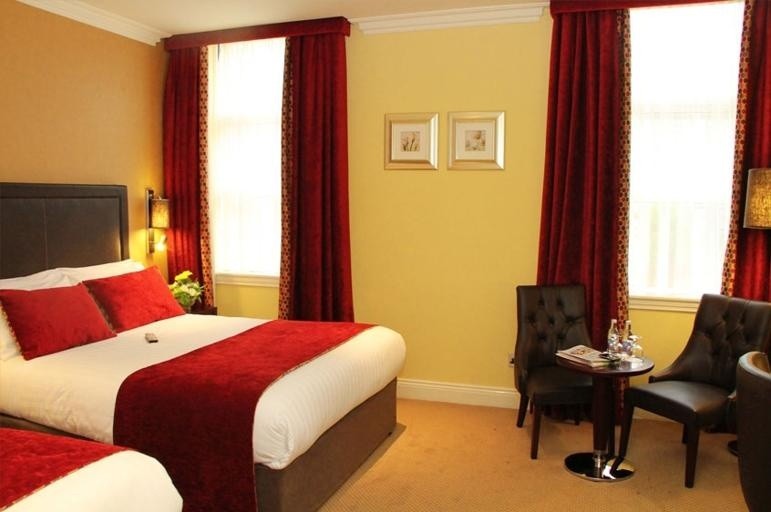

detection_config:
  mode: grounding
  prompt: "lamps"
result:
[744,167,771,231]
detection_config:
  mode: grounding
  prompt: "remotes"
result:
[145,332,158,342]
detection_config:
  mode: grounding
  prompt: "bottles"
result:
[607,319,619,357]
[621,320,634,355]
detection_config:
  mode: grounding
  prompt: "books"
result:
[557,343,622,369]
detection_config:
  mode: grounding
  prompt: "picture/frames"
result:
[383,110,506,172]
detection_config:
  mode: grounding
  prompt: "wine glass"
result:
[627,335,645,362]
